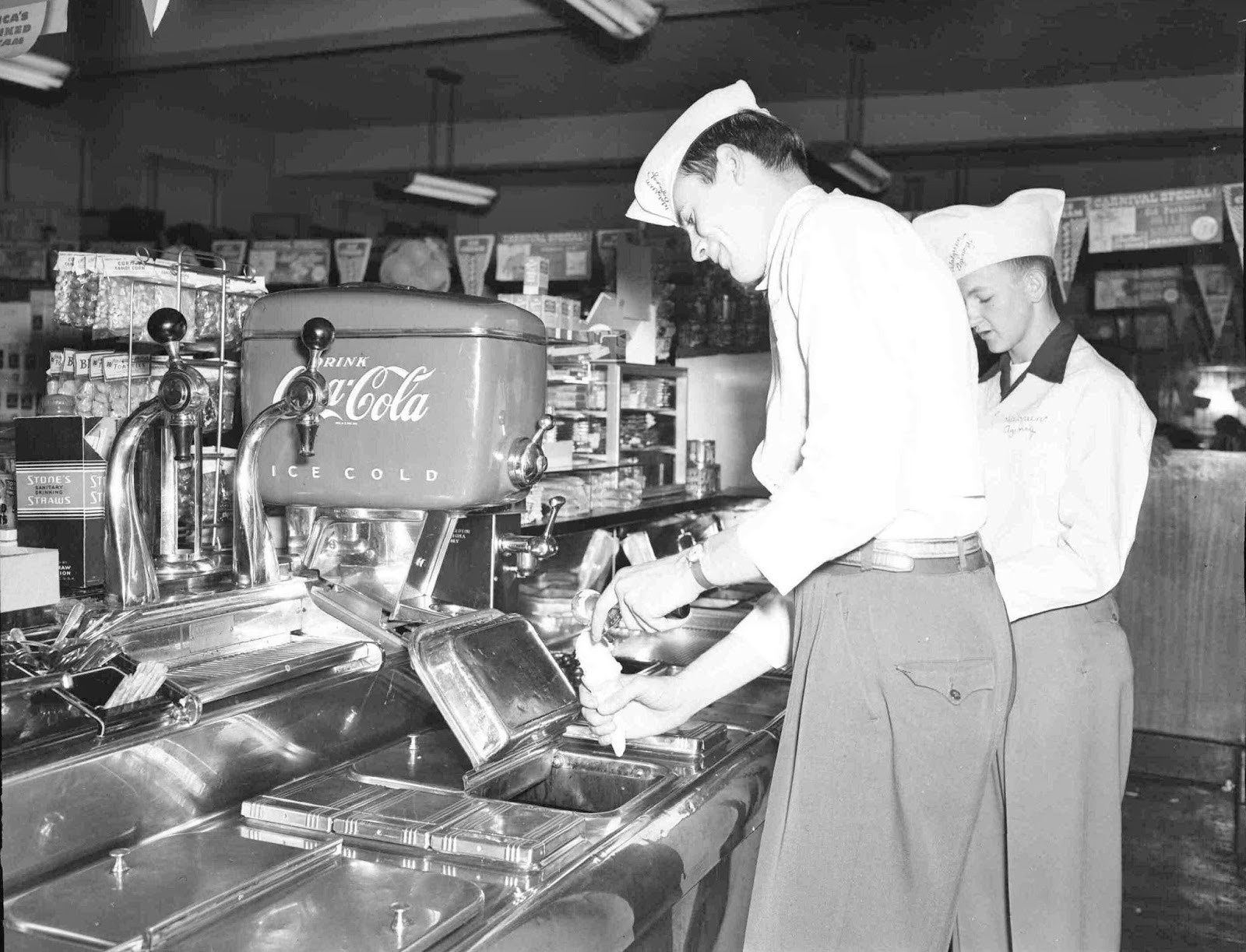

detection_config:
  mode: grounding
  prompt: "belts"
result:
[827,533,981,572]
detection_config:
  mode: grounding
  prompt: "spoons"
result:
[571,588,691,625]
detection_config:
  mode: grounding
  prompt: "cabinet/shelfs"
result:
[545,362,688,498]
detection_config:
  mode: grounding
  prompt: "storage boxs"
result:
[0,546,60,613]
[12,416,127,589]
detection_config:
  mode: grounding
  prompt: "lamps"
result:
[400,67,499,207]
[825,35,892,192]
[565,1,665,41]
[0,50,75,90]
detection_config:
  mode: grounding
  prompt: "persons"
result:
[579,78,1016,952]
[912,187,1158,952]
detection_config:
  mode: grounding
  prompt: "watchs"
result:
[686,543,716,590]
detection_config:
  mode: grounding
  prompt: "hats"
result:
[625,79,774,227]
[913,187,1065,278]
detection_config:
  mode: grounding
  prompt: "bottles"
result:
[686,439,701,497]
[701,441,722,495]
